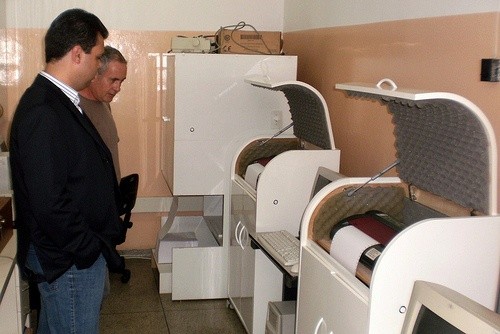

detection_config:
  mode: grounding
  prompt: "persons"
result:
[78,45,127,185]
[9,9,120,334]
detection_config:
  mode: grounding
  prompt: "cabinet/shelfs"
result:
[160,53,298,304]
[226,73,340,334]
[295,76,500,334]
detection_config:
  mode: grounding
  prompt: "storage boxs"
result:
[158,232,199,264]
[215,28,281,54]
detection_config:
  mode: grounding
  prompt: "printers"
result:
[245,155,276,181]
[330,210,408,272]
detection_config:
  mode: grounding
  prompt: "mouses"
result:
[290,264,298,273]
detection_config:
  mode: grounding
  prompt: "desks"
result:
[0,229,23,334]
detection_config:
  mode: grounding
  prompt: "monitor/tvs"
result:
[309,167,348,202]
[401,280,500,334]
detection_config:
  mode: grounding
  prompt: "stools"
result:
[150,248,172,294]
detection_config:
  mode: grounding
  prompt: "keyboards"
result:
[250,229,300,266]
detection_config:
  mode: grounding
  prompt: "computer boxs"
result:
[265,301,295,334]
